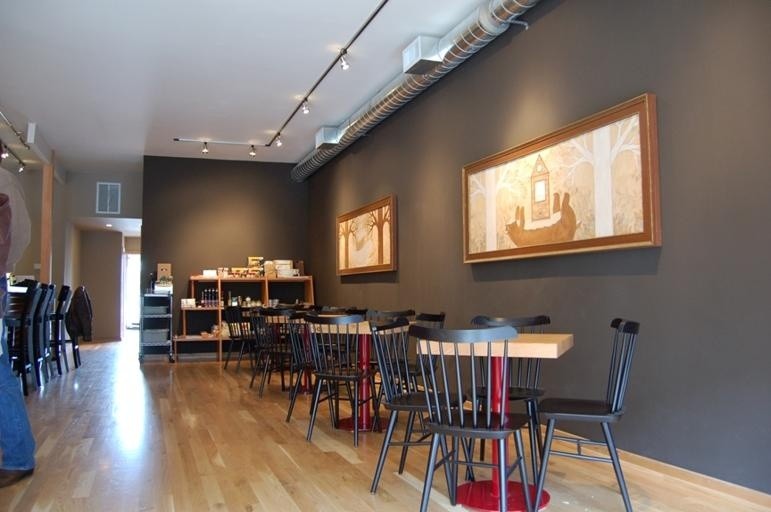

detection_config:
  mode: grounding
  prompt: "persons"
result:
[1,194,37,488]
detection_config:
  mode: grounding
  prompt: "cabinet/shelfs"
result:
[175,274,317,364]
[135,294,173,364]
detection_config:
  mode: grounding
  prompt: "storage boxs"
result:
[141,329,170,344]
[142,305,169,315]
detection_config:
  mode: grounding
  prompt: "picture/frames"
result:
[334,193,398,277]
[460,90,662,264]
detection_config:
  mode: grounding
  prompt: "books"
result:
[200,255,303,338]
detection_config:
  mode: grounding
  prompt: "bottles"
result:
[200,288,218,308]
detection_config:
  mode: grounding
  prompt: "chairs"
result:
[6,272,87,399]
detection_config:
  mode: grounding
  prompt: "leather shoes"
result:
[1,469,33,487]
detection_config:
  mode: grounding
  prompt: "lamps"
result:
[199,139,260,161]
[0,139,26,176]
[273,48,351,149]
